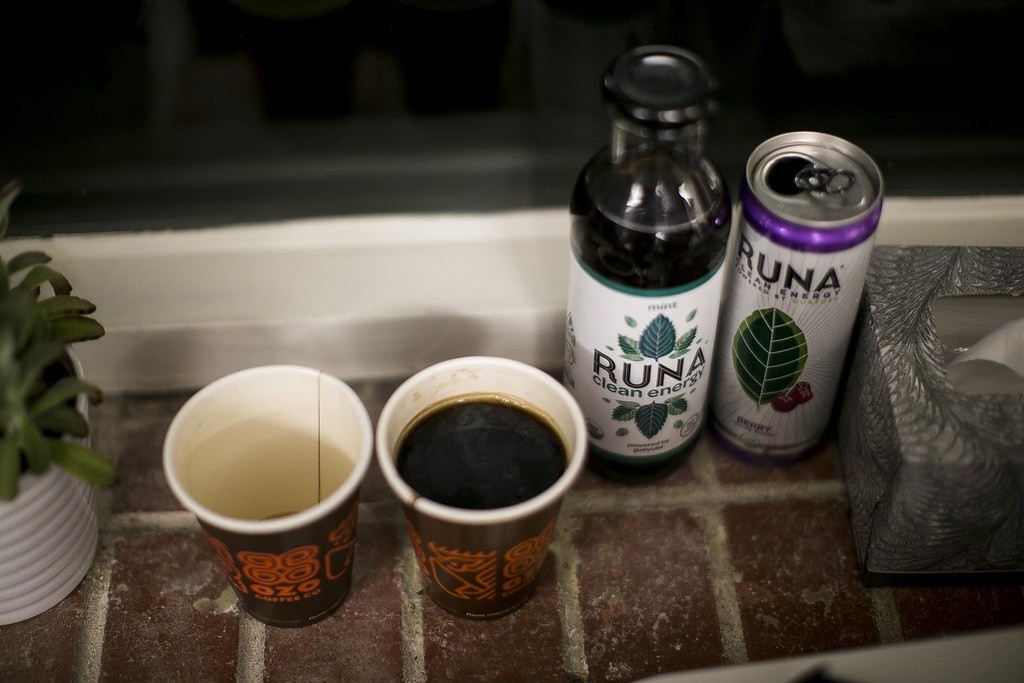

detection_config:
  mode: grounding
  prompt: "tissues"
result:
[833,245,1024,588]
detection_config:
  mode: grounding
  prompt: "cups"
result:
[162,364,374,629]
[374,355,589,622]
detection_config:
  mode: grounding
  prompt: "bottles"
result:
[564,45,735,478]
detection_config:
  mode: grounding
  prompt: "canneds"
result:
[705,131,884,462]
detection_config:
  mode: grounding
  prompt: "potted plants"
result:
[0,178,119,627]
